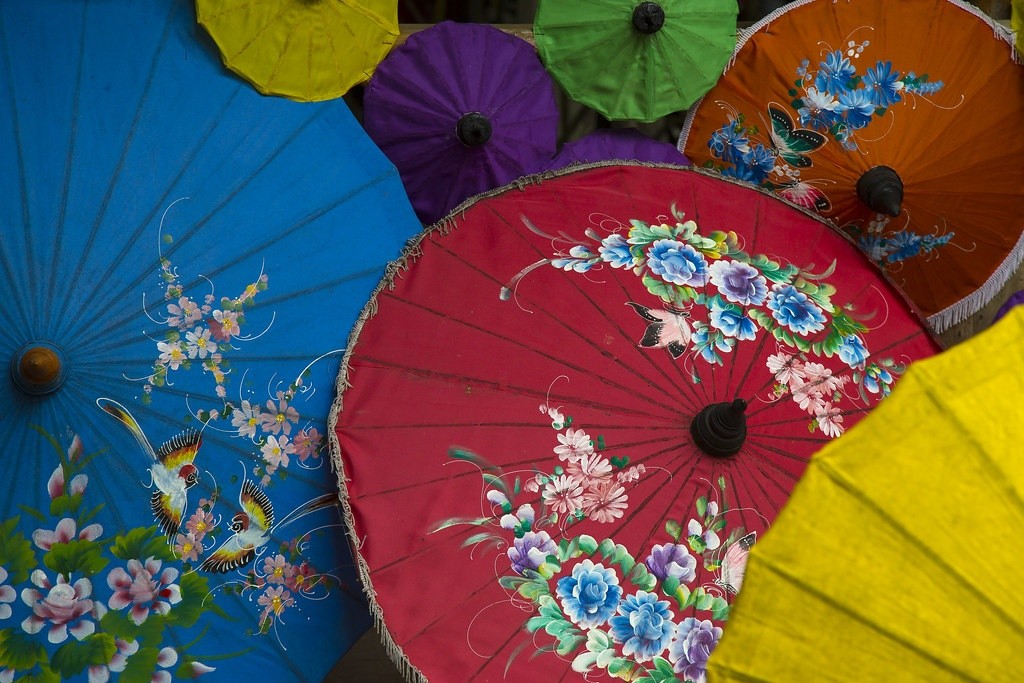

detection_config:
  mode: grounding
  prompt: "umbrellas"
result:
[703,306,1024,683]
[1,1,739,682]
[330,161,954,683]
[681,1,1024,333]
